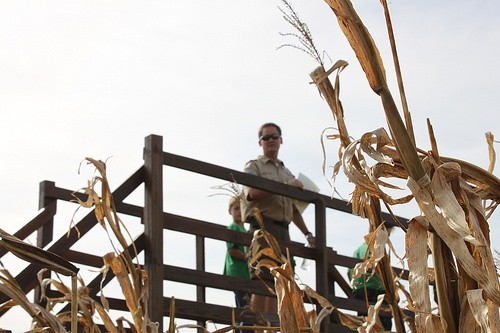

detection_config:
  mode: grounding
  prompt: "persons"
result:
[242,123,316,333]
[347,222,395,331]
[223,196,260,333]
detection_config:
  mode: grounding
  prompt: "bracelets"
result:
[305,232,312,236]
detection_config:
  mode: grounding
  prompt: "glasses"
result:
[260,134,281,141]
